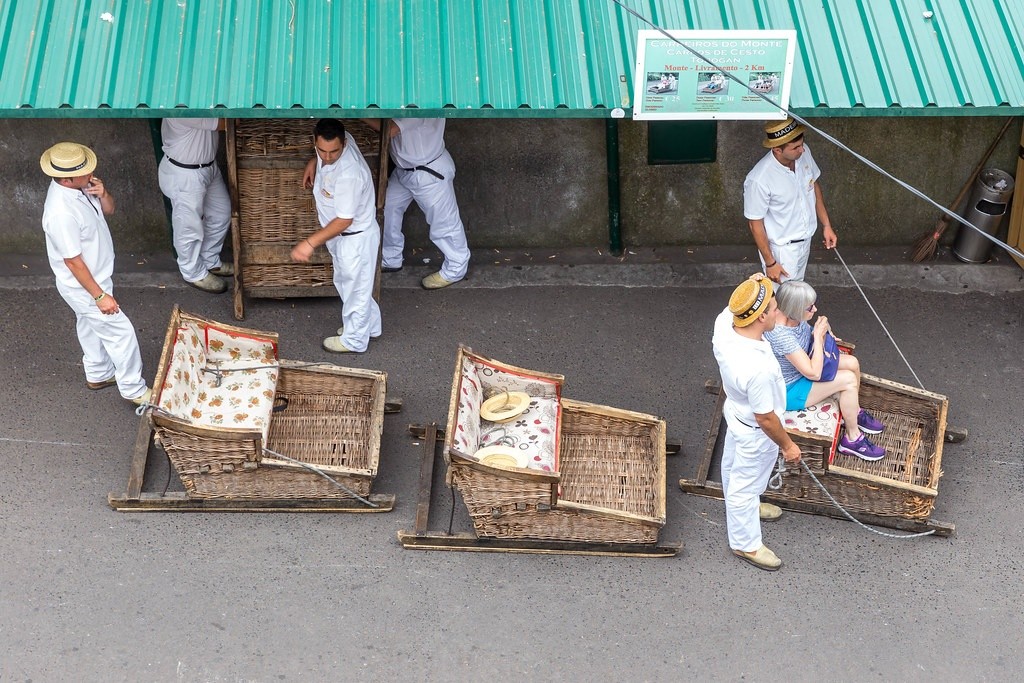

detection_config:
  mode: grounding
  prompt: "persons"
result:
[158,104,240,293]
[763,280,885,460]
[742,117,836,294]
[290,118,381,354]
[360,117,471,289]
[712,272,802,571]
[40,142,152,406]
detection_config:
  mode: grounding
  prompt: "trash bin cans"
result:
[955,169,1015,263]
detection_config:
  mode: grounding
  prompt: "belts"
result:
[165,154,215,169]
[734,413,760,429]
[790,240,805,244]
[340,231,363,236]
[400,153,445,181]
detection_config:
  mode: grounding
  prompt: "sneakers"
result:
[837,433,885,461]
[841,409,884,434]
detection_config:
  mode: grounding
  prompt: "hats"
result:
[39,141,98,178]
[728,276,774,328]
[481,391,530,424]
[762,117,807,148]
[472,445,528,469]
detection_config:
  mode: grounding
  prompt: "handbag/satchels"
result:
[807,324,839,383]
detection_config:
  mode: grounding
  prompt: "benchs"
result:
[145,303,278,460]
[445,341,566,499]
[783,340,855,469]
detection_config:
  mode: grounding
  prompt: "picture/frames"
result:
[633,30,795,121]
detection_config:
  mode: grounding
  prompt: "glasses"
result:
[806,303,813,315]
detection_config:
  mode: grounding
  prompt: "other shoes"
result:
[336,326,381,341]
[323,336,352,354]
[128,388,152,406]
[209,262,234,276]
[421,270,457,289]
[86,375,117,391]
[758,502,782,522]
[185,271,227,294]
[732,545,781,572]
[381,266,402,273]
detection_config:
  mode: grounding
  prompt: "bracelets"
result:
[95,290,105,301]
[306,238,315,249]
[765,260,776,268]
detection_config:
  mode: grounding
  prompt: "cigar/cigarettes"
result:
[92,177,97,179]
